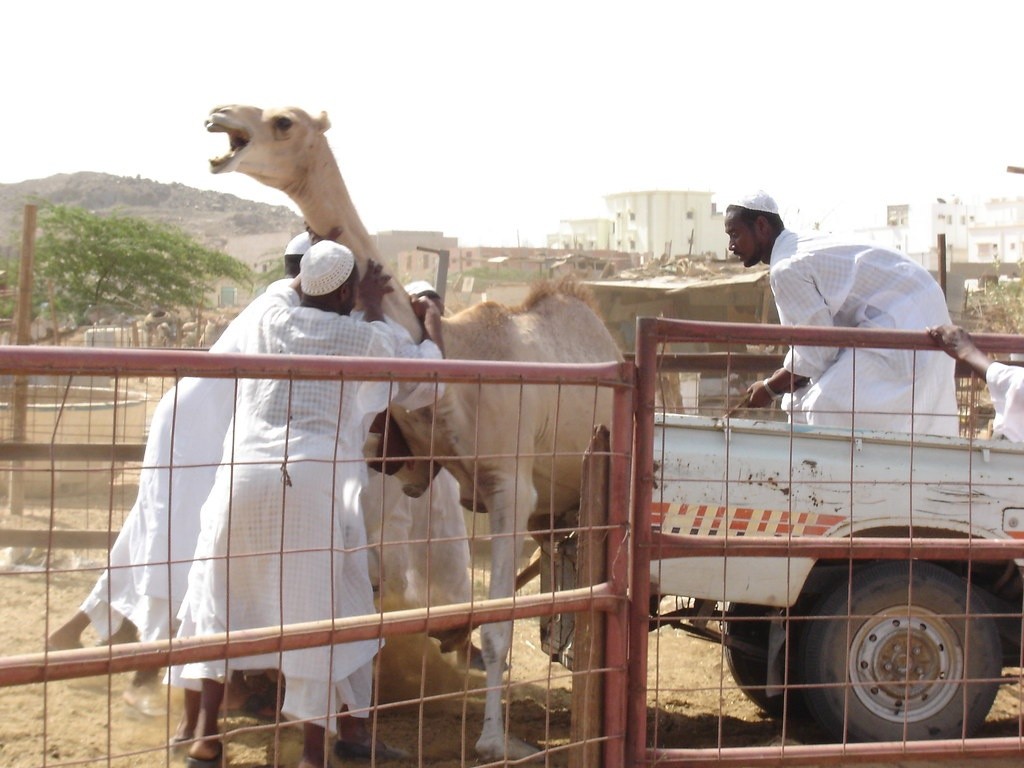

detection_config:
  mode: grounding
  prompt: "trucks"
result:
[651,416,1024,740]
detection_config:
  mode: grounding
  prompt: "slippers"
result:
[185,737,228,768]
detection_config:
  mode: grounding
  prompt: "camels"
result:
[202,102,626,764]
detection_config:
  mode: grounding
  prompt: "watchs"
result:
[764,379,782,400]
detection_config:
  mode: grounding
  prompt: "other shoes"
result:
[336,730,412,763]
[236,687,283,722]
[96,634,139,646]
[124,688,166,717]
[457,649,509,674]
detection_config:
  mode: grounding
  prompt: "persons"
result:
[725,191,958,434]
[46,230,513,768]
[925,324,1024,443]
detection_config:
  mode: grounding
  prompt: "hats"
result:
[728,188,780,215]
[283,231,312,254]
[404,280,435,296]
[299,239,354,295]
